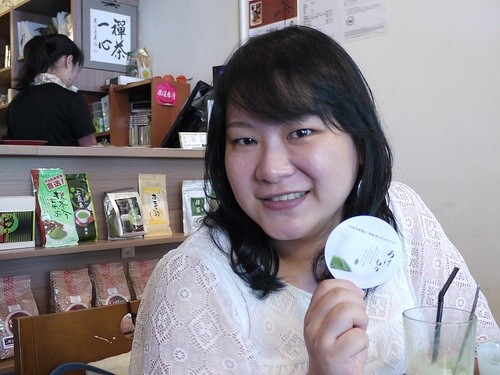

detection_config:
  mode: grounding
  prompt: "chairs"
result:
[12,299,141,375]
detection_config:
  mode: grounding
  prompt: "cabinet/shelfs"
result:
[0,145,207,375]
[0,0,73,110]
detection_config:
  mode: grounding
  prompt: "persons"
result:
[127,25,500,375]
[7,34,98,147]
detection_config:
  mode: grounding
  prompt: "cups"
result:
[402,306,478,375]
[476,340,500,375]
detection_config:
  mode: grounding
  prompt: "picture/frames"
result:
[81,0,140,74]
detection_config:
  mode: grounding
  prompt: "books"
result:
[128,101,151,147]
[5,45,11,68]
